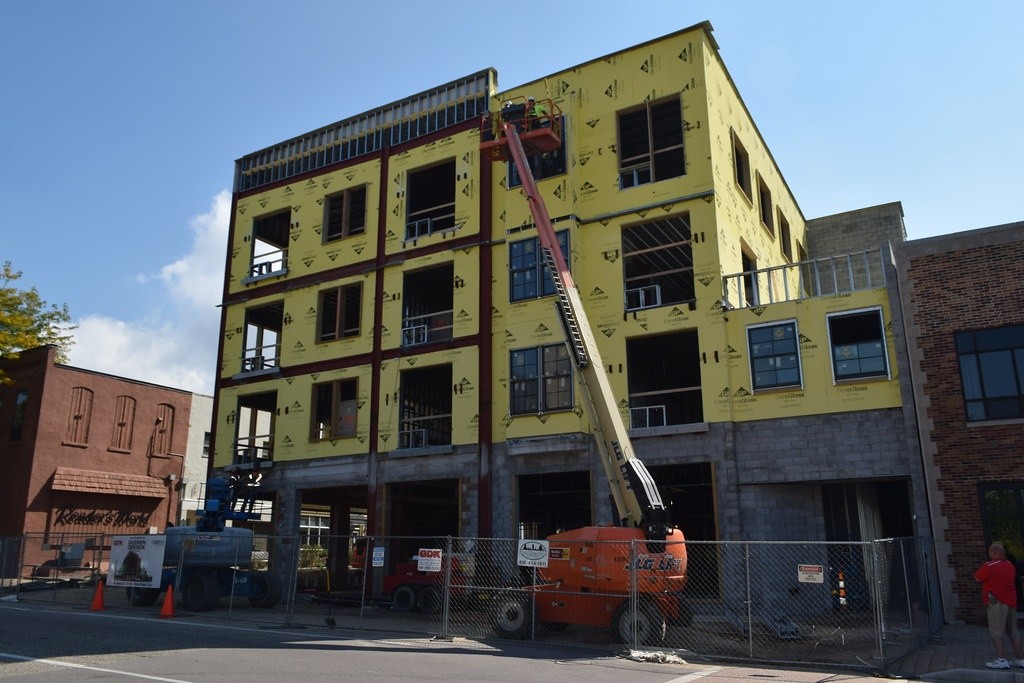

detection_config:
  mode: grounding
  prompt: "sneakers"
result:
[985,657,1010,669]
[1009,658,1024,667]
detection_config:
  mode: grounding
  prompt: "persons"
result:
[976,545,1024,669]
[502,97,541,136]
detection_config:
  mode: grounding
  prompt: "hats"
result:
[988,544,1005,553]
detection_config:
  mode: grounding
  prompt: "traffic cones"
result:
[88,580,109,611]
[159,584,176,618]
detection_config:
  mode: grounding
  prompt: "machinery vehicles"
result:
[126,448,270,612]
[475,89,697,650]
